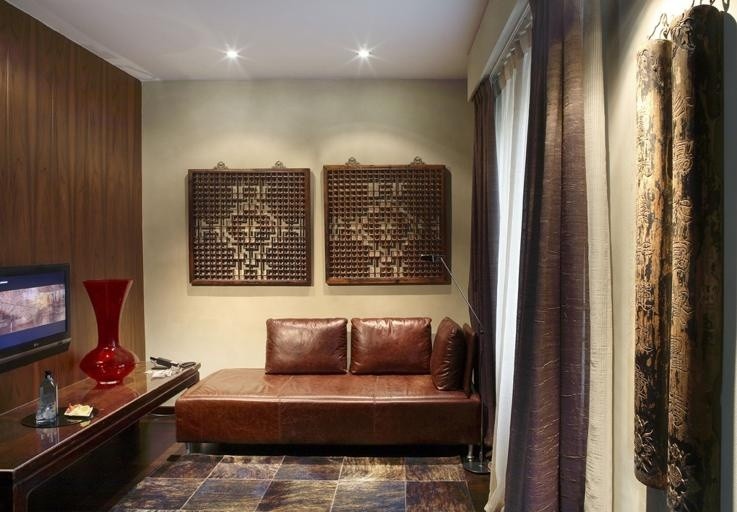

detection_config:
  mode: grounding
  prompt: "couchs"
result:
[176,321,483,462]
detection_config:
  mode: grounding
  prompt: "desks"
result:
[1,360,200,512]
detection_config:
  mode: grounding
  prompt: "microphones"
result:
[421,254,444,263]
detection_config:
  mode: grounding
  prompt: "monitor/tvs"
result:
[0,263,72,374]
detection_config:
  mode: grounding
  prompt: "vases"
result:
[78,279,140,387]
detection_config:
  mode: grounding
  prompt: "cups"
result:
[34,403,56,425]
[36,428,58,446]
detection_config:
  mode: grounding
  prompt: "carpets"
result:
[111,452,475,512]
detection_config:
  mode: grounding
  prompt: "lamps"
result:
[419,253,491,475]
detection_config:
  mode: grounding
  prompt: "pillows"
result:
[430,316,466,391]
[350,317,432,375]
[265,318,348,375]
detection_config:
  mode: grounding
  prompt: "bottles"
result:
[40,370,58,418]
